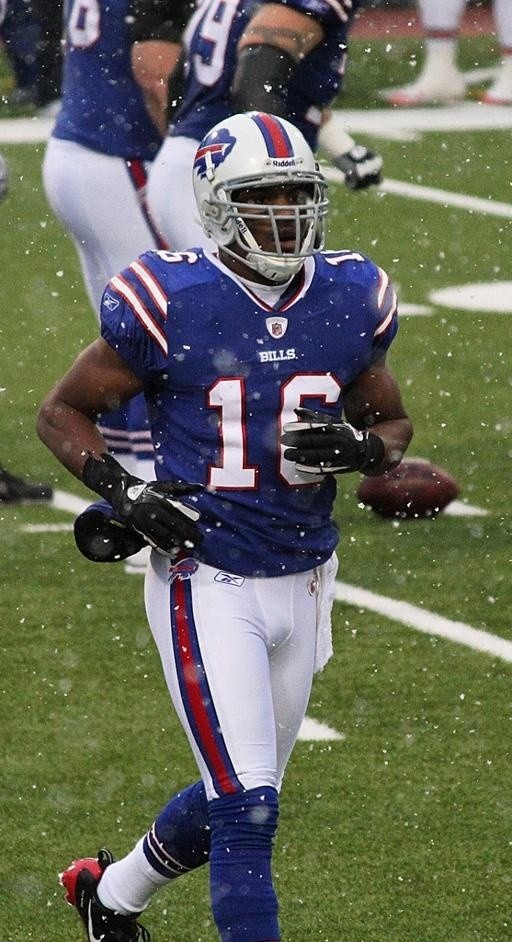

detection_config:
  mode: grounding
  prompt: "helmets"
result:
[192,110,331,281]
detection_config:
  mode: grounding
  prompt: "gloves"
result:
[334,139,386,191]
[278,404,385,480]
[111,473,206,559]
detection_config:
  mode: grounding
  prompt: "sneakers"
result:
[0,466,52,502]
[56,846,154,942]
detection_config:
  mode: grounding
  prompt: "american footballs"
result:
[356,458,460,520]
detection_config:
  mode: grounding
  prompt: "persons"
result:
[36,113,412,942]
[0,0,512,502]
